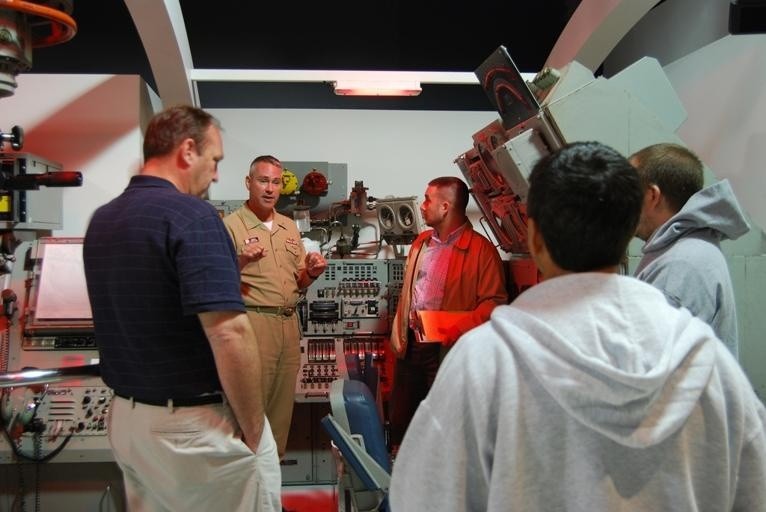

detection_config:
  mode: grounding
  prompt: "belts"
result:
[246,306,296,316]
[113,391,223,406]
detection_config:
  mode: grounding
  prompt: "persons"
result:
[624,143,741,371]
[221,155,327,459]
[388,143,765,510]
[387,178,509,458]
[83,107,282,511]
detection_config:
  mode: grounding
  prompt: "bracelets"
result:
[307,271,319,280]
